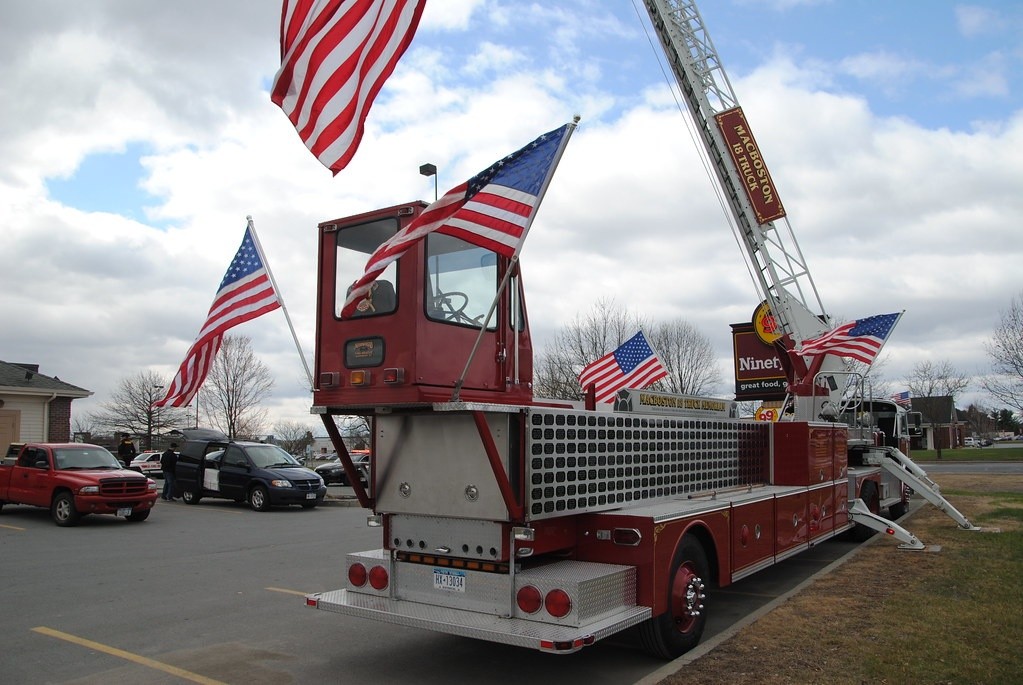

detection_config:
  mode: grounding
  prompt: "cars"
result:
[965,438,974,446]
[315,449,370,487]
[315,452,337,460]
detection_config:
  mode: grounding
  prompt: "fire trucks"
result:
[305,0,976,656]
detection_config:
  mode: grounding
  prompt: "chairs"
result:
[346,280,395,317]
[23,450,40,467]
[64,455,80,466]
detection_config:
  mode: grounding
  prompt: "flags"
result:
[787,312,902,365]
[341,124,568,319]
[895,391,910,404]
[152,226,281,408]
[271,0,427,178]
[578,331,668,404]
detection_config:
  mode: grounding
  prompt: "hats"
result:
[171,443,179,447]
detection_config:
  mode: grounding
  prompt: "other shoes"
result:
[162,496,166,499]
[167,498,176,502]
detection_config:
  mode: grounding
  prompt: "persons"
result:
[118,436,136,469]
[160,443,177,501]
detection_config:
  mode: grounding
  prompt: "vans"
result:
[130,449,180,471]
[169,428,328,511]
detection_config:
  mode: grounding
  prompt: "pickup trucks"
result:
[0,442,158,526]
